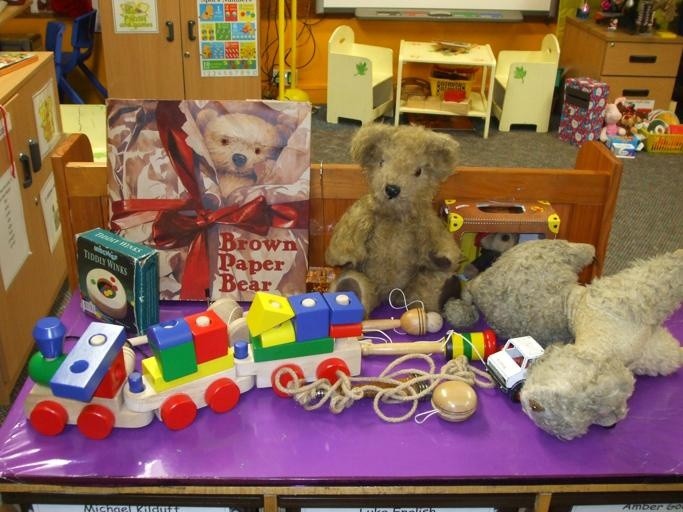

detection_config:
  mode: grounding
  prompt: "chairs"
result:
[325,24,394,127]
[62,9,108,98]
[491,33,561,133]
[44,21,85,105]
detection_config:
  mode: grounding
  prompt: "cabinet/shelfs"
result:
[395,38,497,138]
[0,49,69,409]
[97,0,262,100]
[0,285,683,512]
[559,14,683,112]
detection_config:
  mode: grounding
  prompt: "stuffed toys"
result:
[107,97,308,294]
[468,238,683,441]
[325,123,465,322]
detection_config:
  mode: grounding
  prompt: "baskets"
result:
[427,64,475,99]
[639,128,682,154]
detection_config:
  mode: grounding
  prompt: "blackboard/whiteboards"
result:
[316,0,559,21]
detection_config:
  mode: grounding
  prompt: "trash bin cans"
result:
[557,78,610,148]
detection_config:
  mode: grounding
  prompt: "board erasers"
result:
[427,11,454,17]
